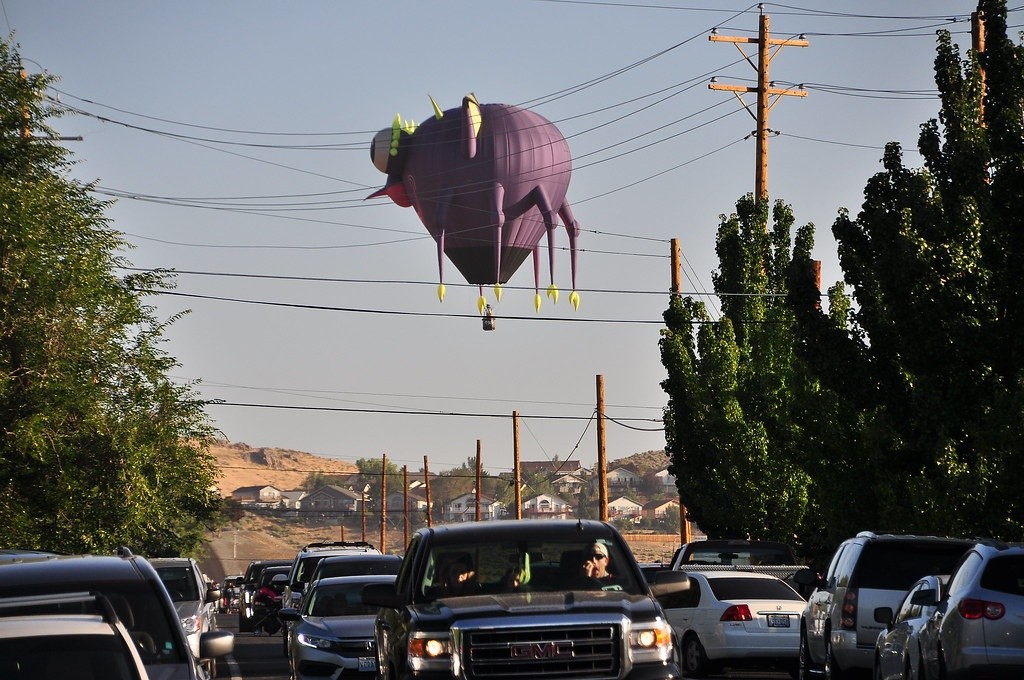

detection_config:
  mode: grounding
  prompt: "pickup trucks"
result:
[670,539,809,594]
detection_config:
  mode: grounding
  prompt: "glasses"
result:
[584,554,607,560]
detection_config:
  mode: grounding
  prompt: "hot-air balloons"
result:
[364,92,580,331]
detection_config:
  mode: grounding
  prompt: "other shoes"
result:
[227,609,229,614]
[229,611,231,614]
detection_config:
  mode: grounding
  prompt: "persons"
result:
[432,552,487,600]
[211,582,235,614]
[498,565,533,593]
[485,305,492,319]
[316,586,382,614]
[566,541,627,590]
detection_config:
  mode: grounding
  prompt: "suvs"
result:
[0,546,234,680]
[220,519,690,680]
[918,540,1024,680]
[799,530,1010,680]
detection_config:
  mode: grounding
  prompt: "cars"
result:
[874,574,952,680]
[642,567,808,680]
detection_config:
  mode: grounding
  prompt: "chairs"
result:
[428,552,480,595]
